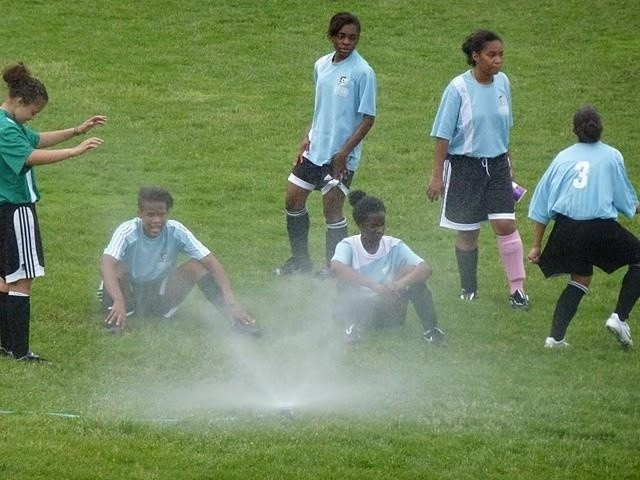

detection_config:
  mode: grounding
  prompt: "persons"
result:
[329,188,455,352]
[275,10,378,282]
[527,103,640,351]
[0,58,109,368]
[95,184,265,342]
[424,26,530,313]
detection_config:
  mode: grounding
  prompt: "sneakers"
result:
[422,326,446,344]
[273,254,314,274]
[345,325,360,344]
[508,289,530,311]
[605,312,633,347]
[15,351,53,365]
[545,336,570,349]
[1,347,13,357]
[458,289,478,303]
